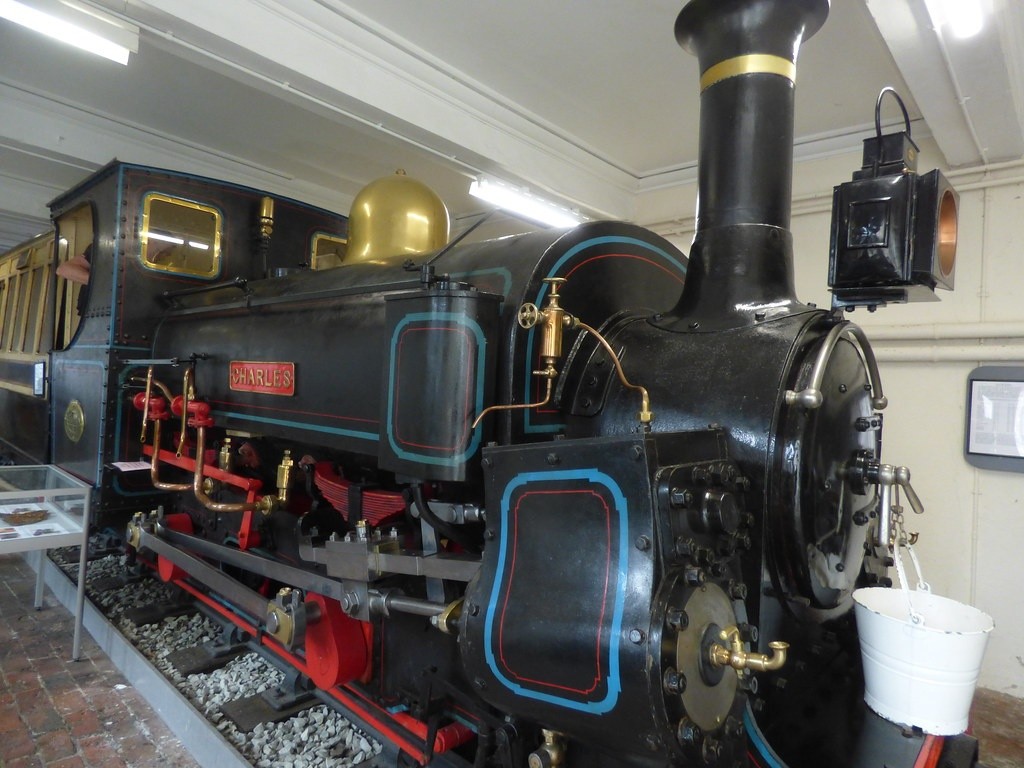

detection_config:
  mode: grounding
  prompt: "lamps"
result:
[469,180,586,229]
[0,0,140,66]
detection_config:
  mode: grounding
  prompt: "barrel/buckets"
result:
[851,537,995,736]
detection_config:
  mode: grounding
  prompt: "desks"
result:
[0,465,94,661]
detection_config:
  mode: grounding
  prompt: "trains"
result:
[0,1,996,768]
